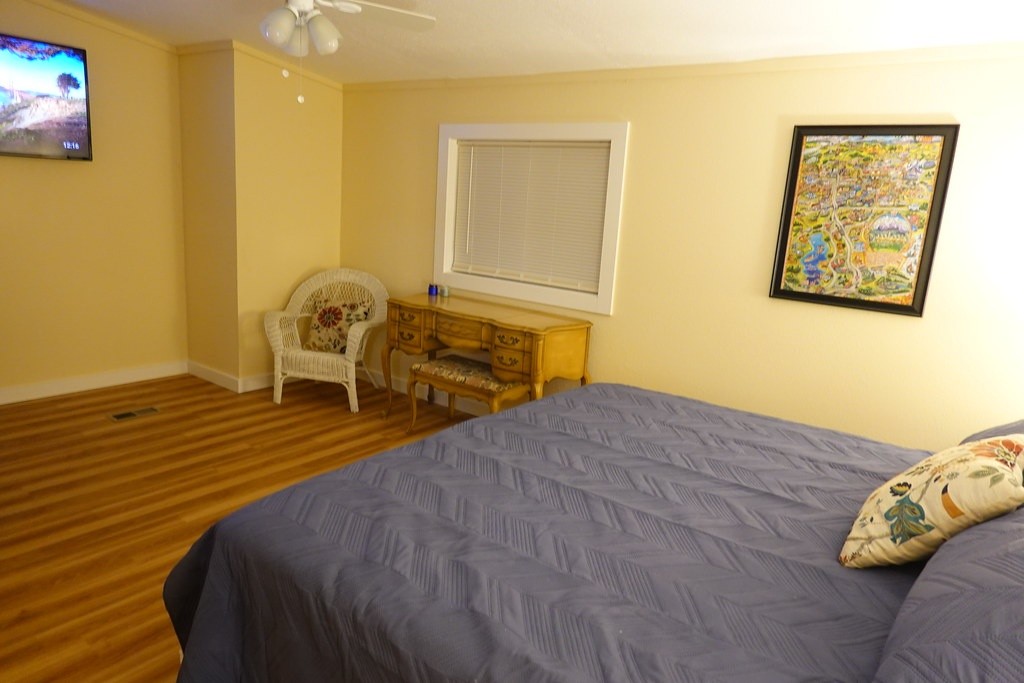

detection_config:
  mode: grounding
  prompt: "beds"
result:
[162,383,930,683]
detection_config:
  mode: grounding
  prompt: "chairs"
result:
[264,268,391,413]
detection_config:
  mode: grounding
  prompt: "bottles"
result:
[429,283,449,298]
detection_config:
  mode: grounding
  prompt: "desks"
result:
[380,293,593,420]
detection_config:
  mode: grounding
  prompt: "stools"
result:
[405,354,531,434]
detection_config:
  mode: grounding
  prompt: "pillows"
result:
[875,507,1024,683]
[304,299,374,354]
[958,420,1024,445]
[838,433,1024,570]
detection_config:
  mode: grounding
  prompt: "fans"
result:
[286,0,436,21]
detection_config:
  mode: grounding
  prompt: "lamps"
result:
[258,6,344,104]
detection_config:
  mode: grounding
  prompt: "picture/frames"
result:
[768,124,962,319]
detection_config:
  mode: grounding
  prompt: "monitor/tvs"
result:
[0,33,93,162]
